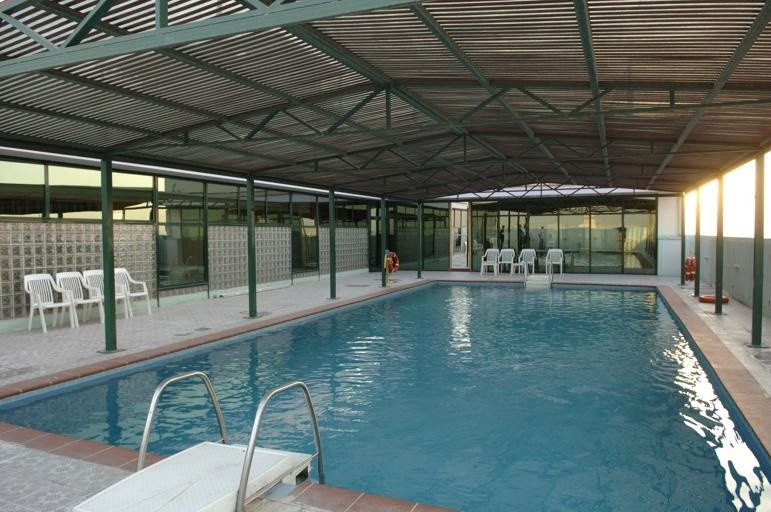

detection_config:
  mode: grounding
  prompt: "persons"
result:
[519,224,524,252]
[500,225,504,249]
[538,226,544,249]
[523,223,526,249]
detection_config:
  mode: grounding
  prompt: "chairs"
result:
[544,248,564,275]
[22,267,153,333]
[479,246,537,274]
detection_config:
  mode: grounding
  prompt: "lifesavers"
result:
[686,256,697,281]
[699,294,728,303]
[387,252,399,272]
